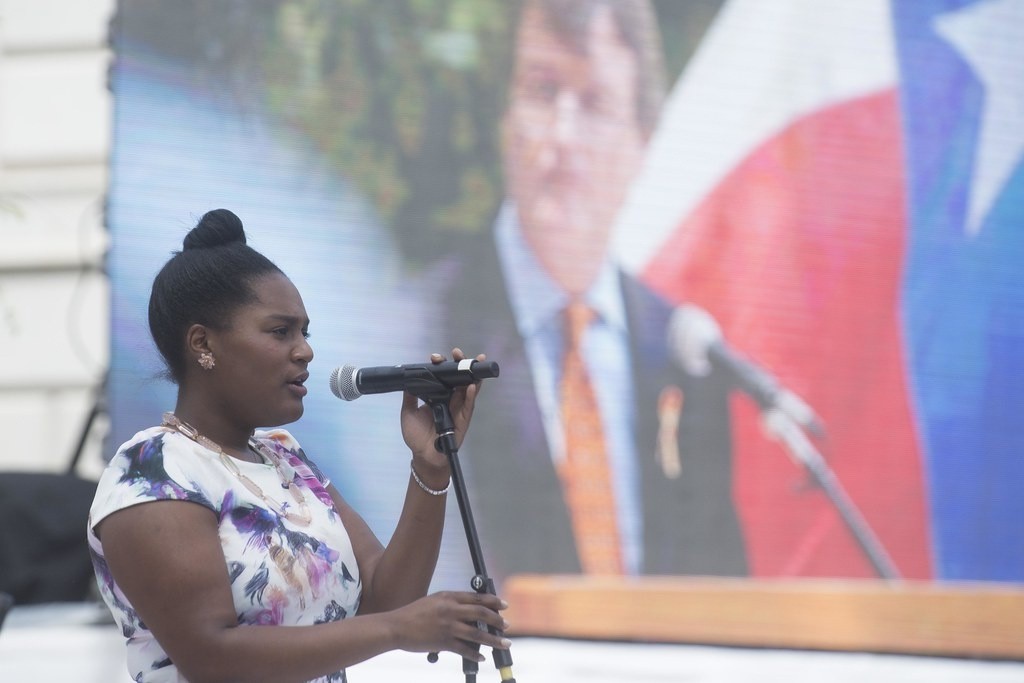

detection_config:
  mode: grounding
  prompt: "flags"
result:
[606,0,1024,582]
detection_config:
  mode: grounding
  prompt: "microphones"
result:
[330,357,500,402]
[667,302,822,436]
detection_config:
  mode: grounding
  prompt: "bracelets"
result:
[410,460,451,496]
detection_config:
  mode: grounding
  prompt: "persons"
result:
[87,208,512,683]
[412,0,748,575]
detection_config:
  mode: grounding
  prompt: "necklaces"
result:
[161,411,311,525]
